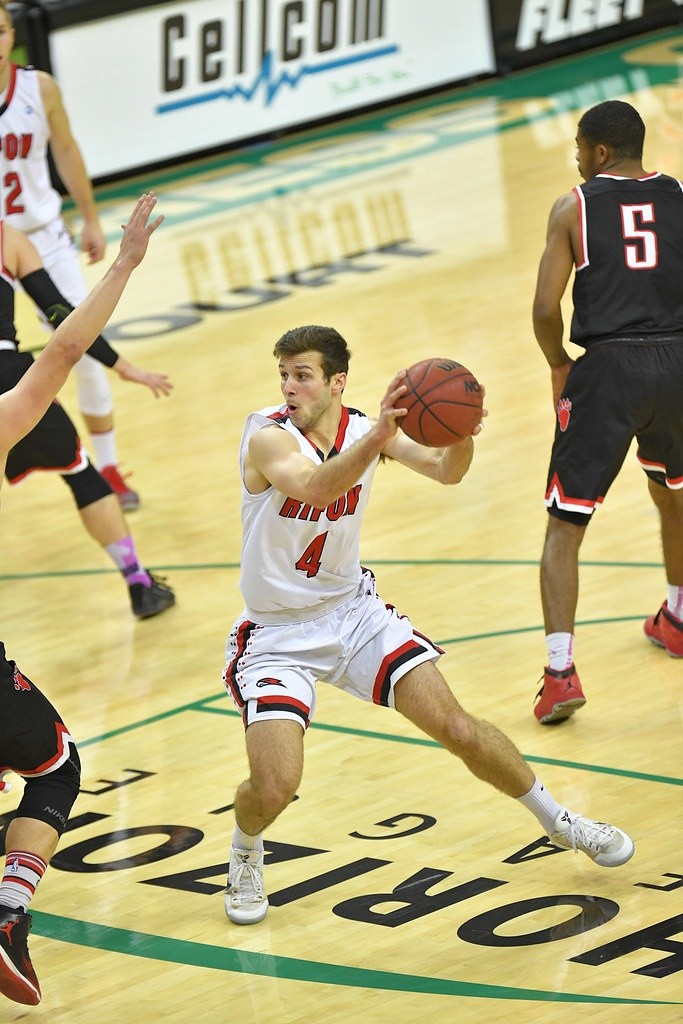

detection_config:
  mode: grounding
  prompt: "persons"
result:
[222,323,633,924]
[0,191,166,1005]
[0,0,177,619]
[532,101,683,724]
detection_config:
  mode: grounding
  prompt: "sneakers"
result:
[534,665,586,724]
[100,466,140,511]
[549,808,635,867]
[0,905,42,1006]
[225,842,268,925]
[643,600,683,657]
[130,568,174,618]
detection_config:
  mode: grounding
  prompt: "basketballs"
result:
[393,358,484,449]
